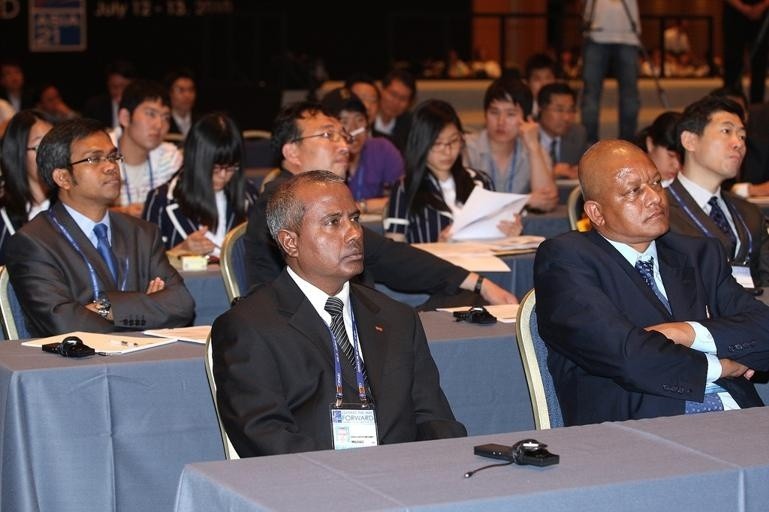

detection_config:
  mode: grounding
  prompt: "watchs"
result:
[95,296,111,318]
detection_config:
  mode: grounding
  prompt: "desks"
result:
[0,78,769,512]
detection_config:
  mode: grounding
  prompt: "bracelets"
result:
[474,275,483,294]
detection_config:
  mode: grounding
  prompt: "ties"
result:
[94,223,121,282]
[634,255,723,414]
[325,297,372,400]
[709,194,737,244]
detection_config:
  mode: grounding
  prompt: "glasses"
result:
[431,137,467,152]
[214,164,241,176]
[71,151,123,172]
[295,130,353,146]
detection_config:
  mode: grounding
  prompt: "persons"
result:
[211,170,468,460]
[308,55,587,209]
[722,2,767,104]
[381,99,524,243]
[582,2,642,145]
[635,96,769,293]
[3,63,255,258]
[3,117,196,338]
[535,139,769,427]
[524,54,768,196]
[242,102,518,312]
